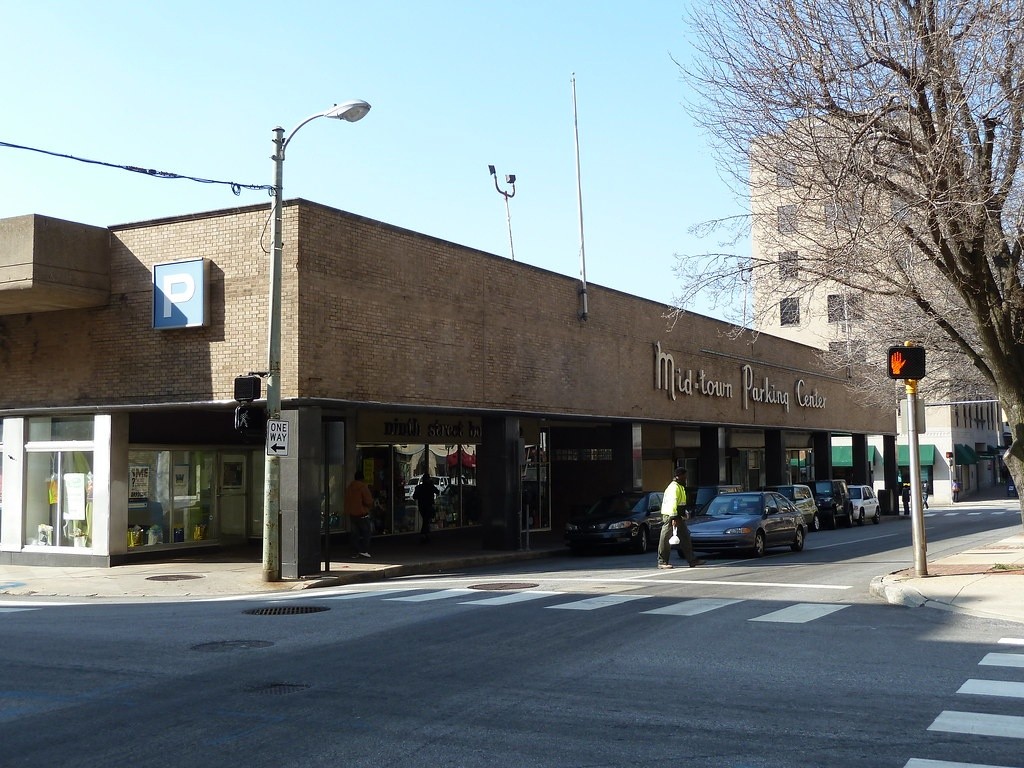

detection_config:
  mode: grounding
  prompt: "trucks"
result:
[404,474,450,504]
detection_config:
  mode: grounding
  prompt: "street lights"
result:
[262,100,371,581]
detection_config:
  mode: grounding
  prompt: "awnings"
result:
[898,445,934,466]
[955,444,980,465]
[832,446,875,466]
[791,459,805,466]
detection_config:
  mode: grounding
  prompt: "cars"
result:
[563,491,689,553]
[678,491,808,558]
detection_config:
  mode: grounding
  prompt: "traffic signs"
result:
[267,419,289,456]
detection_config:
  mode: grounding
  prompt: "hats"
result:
[673,467,687,477]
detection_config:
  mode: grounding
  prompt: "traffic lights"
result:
[233,375,261,401]
[946,452,953,458]
[234,407,250,430]
[886,346,925,380]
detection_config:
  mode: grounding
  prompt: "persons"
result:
[952,479,961,502]
[413,474,440,538]
[657,467,707,569]
[922,480,931,509]
[902,485,910,515]
[344,470,373,559]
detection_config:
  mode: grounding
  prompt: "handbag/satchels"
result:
[952,487,960,492]
[669,525,680,544]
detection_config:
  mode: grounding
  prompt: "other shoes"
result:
[658,562,673,569]
[688,560,707,568]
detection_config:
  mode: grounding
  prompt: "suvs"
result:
[692,479,881,532]
